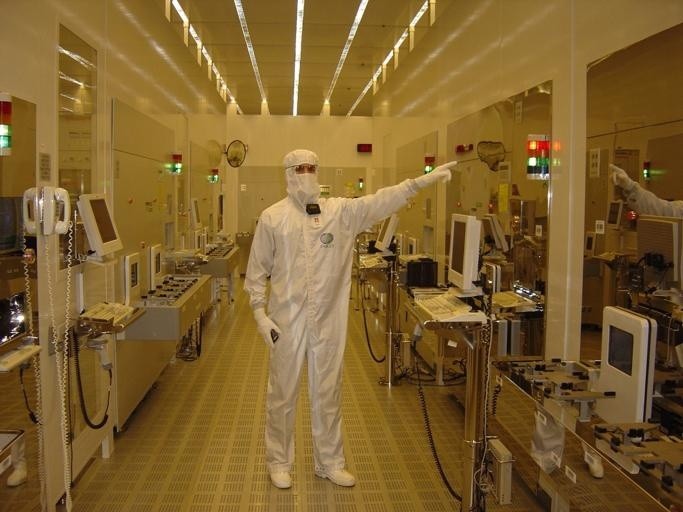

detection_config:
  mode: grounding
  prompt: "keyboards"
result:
[418,292,473,319]
[80,302,135,326]
[361,255,379,268]
[592,254,616,262]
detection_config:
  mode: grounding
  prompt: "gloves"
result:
[415,161,458,189]
[608,164,633,191]
[252,307,281,350]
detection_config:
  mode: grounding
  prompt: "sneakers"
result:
[270,471,292,489]
[315,467,356,487]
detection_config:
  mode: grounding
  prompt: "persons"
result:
[608,163,683,222]
[241,149,458,486]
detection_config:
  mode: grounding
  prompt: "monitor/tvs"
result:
[605,199,624,229]
[191,198,203,230]
[374,213,399,258]
[481,214,509,253]
[76,194,124,263]
[0,196,23,255]
[447,213,483,298]
[636,214,683,300]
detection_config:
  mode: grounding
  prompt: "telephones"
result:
[42,187,72,235]
[23,188,35,236]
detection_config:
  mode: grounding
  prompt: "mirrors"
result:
[442,76,553,309]
[575,20,683,416]
[108,96,215,257]
[51,9,106,282]
[395,131,437,261]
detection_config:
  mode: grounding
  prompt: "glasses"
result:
[287,165,317,173]
